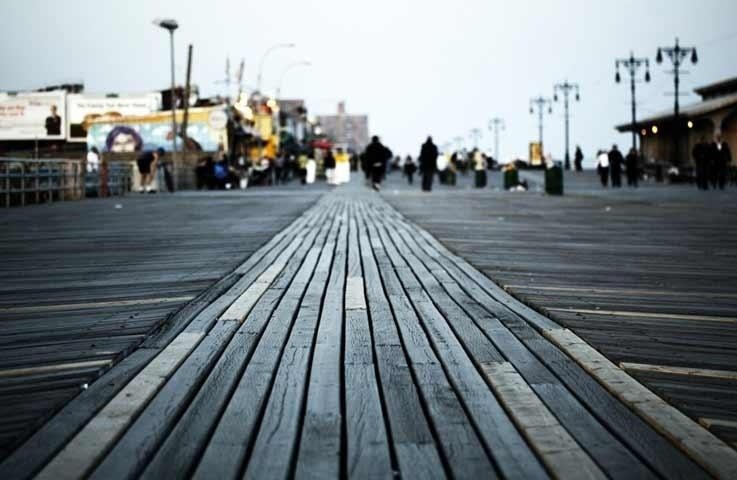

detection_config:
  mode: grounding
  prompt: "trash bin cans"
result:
[475,170,486,188]
[545,166,563,195]
[504,170,517,190]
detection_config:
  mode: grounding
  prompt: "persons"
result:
[45,105,61,136]
[87,146,100,173]
[574,146,586,171]
[596,134,737,194]
[136,147,165,193]
[202,113,534,194]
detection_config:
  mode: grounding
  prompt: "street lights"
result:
[614,50,650,167]
[273,59,312,100]
[158,19,178,192]
[529,94,552,166]
[655,37,698,172]
[436,116,506,167]
[553,77,580,171]
[254,41,296,90]
